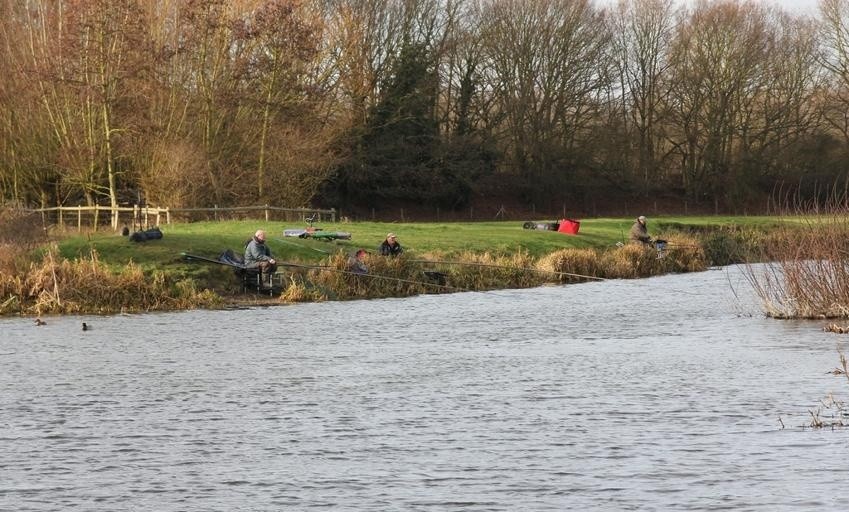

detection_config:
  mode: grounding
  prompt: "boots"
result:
[261,273,273,288]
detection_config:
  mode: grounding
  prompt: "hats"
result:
[387,233,396,238]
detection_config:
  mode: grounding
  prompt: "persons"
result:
[348,248,370,288]
[243,230,276,287]
[122,221,129,236]
[379,232,403,256]
[629,215,654,248]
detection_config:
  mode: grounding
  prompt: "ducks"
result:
[80,323,94,330]
[34,318,46,325]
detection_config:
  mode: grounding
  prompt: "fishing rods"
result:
[276,262,513,297]
[181,253,278,275]
[394,256,701,296]
